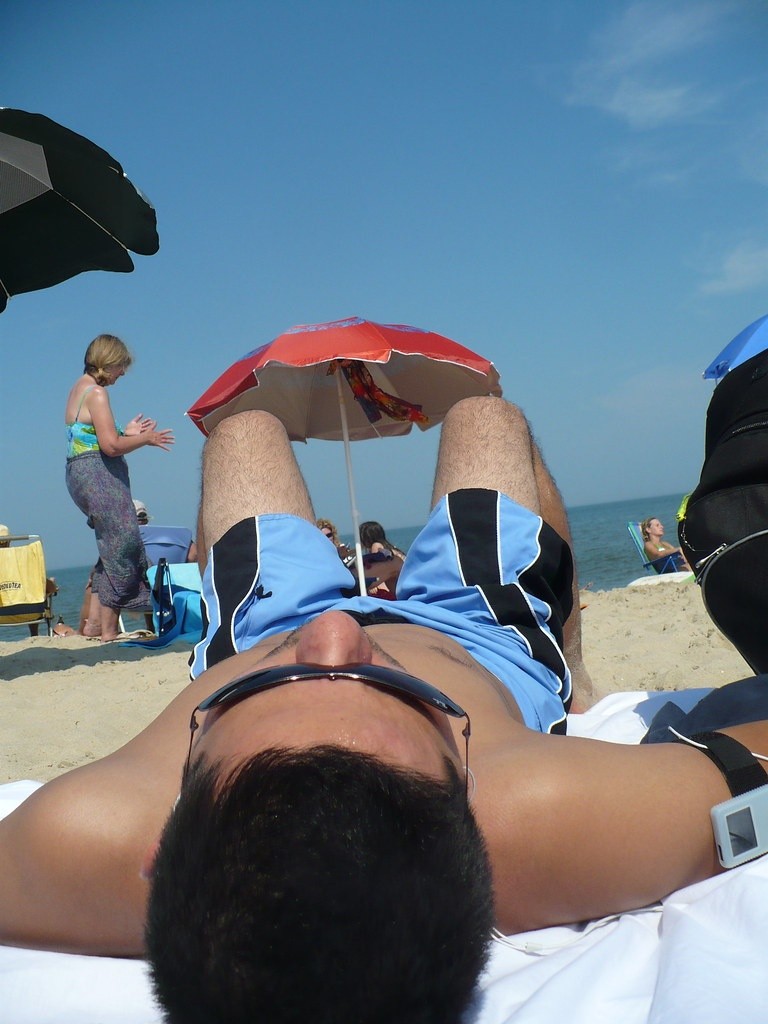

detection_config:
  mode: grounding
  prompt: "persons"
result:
[78,495,198,636]
[360,521,408,599]
[639,516,693,573]
[3,390,768,1024]
[0,524,60,636]
[316,519,351,562]
[63,331,177,644]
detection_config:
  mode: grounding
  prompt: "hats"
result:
[133,499,148,519]
[0,525,10,537]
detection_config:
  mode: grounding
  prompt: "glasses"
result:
[326,532,334,538]
[179,659,474,822]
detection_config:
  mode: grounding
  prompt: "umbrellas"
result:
[698,313,767,382]
[3,103,162,313]
[187,320,510,603]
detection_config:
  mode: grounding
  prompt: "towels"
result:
[0,779,44,819]
[116,584,203,650]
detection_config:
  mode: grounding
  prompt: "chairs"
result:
[115,524,193,634]
[0,535,59,642]
[627,520,686,577]
